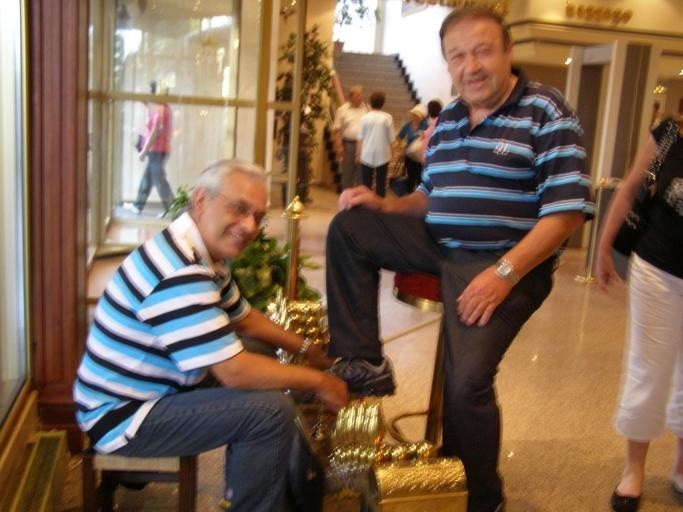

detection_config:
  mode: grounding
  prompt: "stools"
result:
[84,449,196,511]
[392,273,449,448]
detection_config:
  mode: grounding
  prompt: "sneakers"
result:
[290,356,395,404]
[123,203,141,215]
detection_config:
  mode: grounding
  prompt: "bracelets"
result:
[298,336,315,357]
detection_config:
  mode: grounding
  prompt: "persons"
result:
[333,82,373,193]
[356,91,399,199]
[395,101,429,192]
[119,80,180,219]
[291,2,593,512]
[72,155,351,512]
[418,101,444,163]
[589,98,682,512]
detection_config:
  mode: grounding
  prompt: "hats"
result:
[410,104,428,121]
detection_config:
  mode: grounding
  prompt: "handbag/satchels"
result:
[611,184,652,258]
[405,137,421,164]
[389,174,415,195]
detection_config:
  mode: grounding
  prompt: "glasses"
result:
[214,192,269,224]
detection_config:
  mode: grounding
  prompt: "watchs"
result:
[497,258,520,287]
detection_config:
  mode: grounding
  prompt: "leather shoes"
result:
[610,480,642,512]
